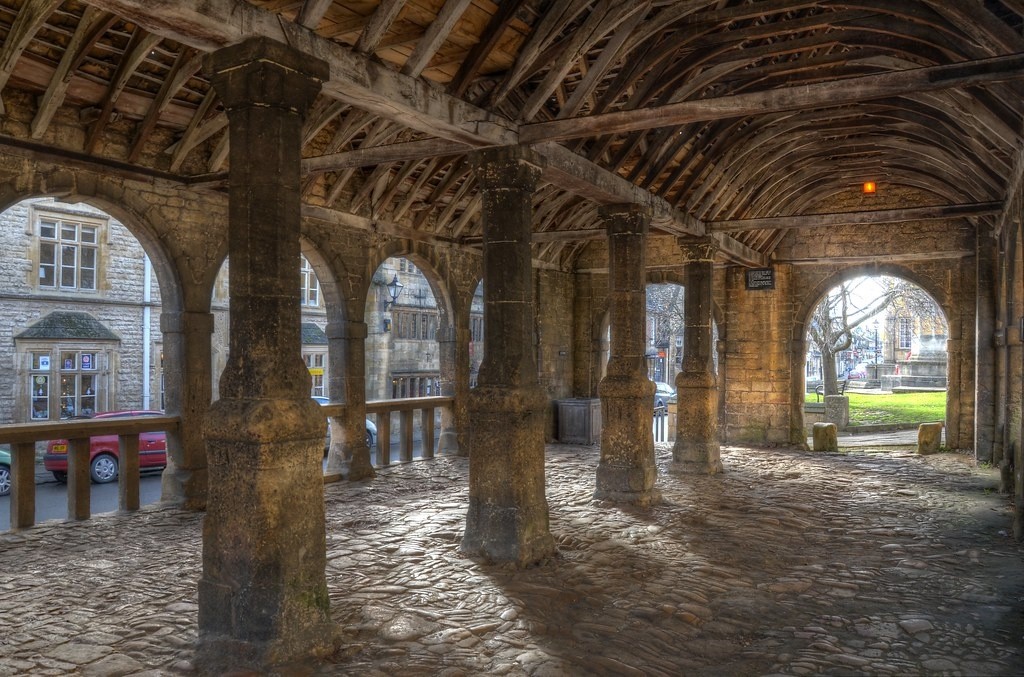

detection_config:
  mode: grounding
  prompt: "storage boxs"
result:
[556,395,602,444]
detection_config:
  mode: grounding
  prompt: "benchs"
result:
[807,380,819,392]
[815,379,846,402]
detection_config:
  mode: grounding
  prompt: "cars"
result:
[654,381,675,412]
[0,451,12,496]
[848,368,866,379]
[311,396,378,450]
[45,411,165,483]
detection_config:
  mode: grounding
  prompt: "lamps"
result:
[384,272,404,312]
[863,181,875,193]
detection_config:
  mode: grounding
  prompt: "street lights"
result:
[874,319,878,380]
